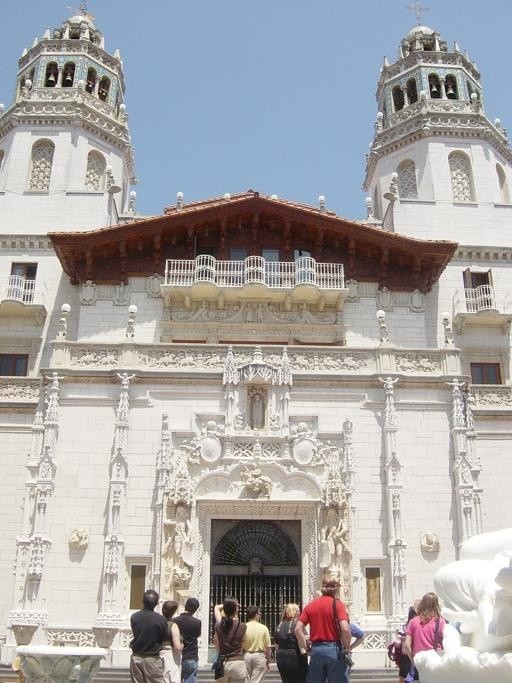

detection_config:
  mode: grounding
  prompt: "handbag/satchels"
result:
[212,662,224,680]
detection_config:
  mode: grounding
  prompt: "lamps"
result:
[388,169,399,193]
[439,309,456,350]
[56,302,71,339]
[176,191,184,206]
[271,193,278,199]
[223,191,232,200]
[318,193,325,208]
[124,305,138,338]
[108,163,117,187]
[126,191,138,214]
[374,307,392,347]
[365,193,374,222]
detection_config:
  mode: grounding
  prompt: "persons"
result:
[165,505,195,584]
[158,600,184,683]
[294,576,352,682]
[321,508,350,560]
[213,597,247,683]
[273,603,309,683]
[252,394,262,430]
[398,607,417,683]
[129,590,168,683]
[404,592,444,679]
[341,612,365,654]
[241,606,272,683]
[172,598,201,683]
